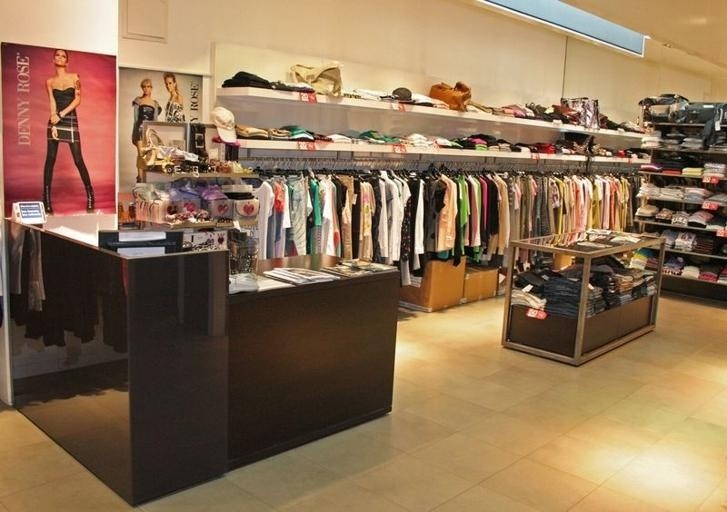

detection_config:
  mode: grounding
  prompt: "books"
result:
[256,258,399,293]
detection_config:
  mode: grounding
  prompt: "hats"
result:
[393,87,415,103]
[210,107,237,145]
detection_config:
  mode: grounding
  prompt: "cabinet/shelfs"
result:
[134,119,258,261]
[501,229,666,367]
[633,121,727,302]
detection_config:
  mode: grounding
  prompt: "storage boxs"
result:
[399,255,508,313]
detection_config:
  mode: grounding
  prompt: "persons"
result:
[184,233,225,252]
[42,49,95,214]
[165,73,186,123]
[131,79,162,183]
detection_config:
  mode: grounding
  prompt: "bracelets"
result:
[56,112,62,118]
[51,125,56,129]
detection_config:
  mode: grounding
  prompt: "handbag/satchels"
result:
[429,82,471,113]
[640,93,727,125]
[289,61,342,97]
[561,96,602,131]
